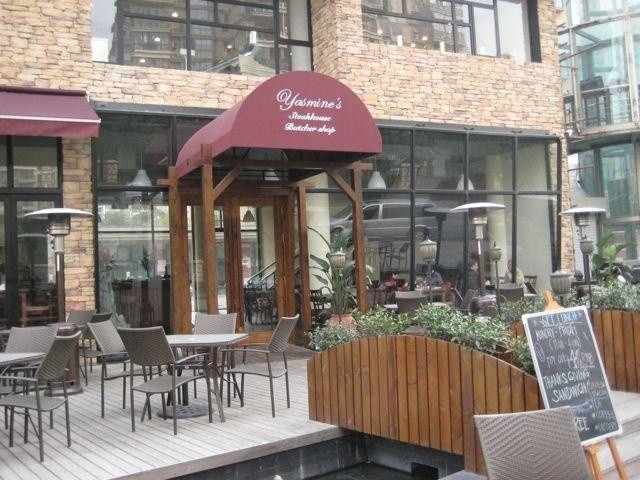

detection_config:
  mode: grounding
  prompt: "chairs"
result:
[373,261,639,320]
[2,330,83,462]
[1,308,249,435]
[220,314,300,417]
[469,403,594,480]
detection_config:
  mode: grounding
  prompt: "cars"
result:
[598,257,640,286]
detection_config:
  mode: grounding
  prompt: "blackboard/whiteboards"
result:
[521,305,622,447]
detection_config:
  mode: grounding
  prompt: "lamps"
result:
[242,206,256,223]
[456,140,476,191]
[130,132,155,188]
[367,152,388,191]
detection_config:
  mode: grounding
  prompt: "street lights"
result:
[422,204,452,268]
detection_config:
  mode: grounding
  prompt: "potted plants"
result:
[308,220,363,330]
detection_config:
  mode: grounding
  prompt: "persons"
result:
[455,259,481,308]
[504,260,529,294]
[379,269,408,293]
[421,264,444,287]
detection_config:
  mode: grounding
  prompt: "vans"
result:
[331,197,438,250]
